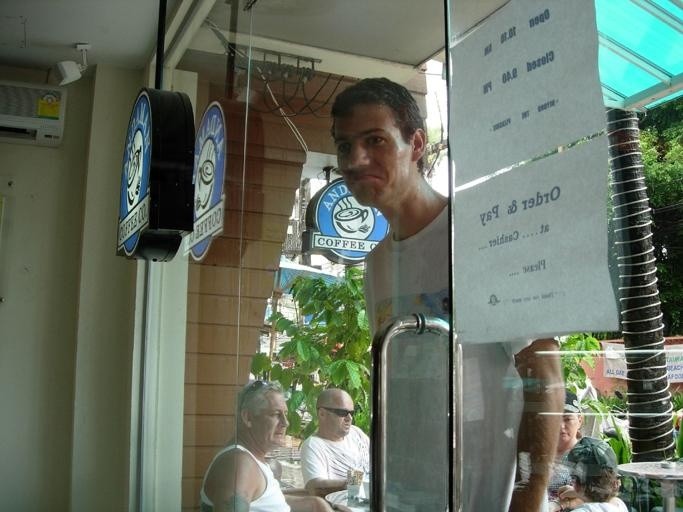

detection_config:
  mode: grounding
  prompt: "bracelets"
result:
[550,499,565,512]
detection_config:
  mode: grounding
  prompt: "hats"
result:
[563,387,582,413]
[562,437,618,484]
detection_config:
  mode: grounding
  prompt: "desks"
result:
[616,459,683,512]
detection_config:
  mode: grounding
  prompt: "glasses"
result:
[240,380,281,409]
[318,407,355,417]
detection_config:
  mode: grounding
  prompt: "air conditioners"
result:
[0,78,69,149]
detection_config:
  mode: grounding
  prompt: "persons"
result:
[298,387,371,496]
[546,386,585,511]
[199,378,335,511]
[566,435,630,511]
[330,76,569,512]
